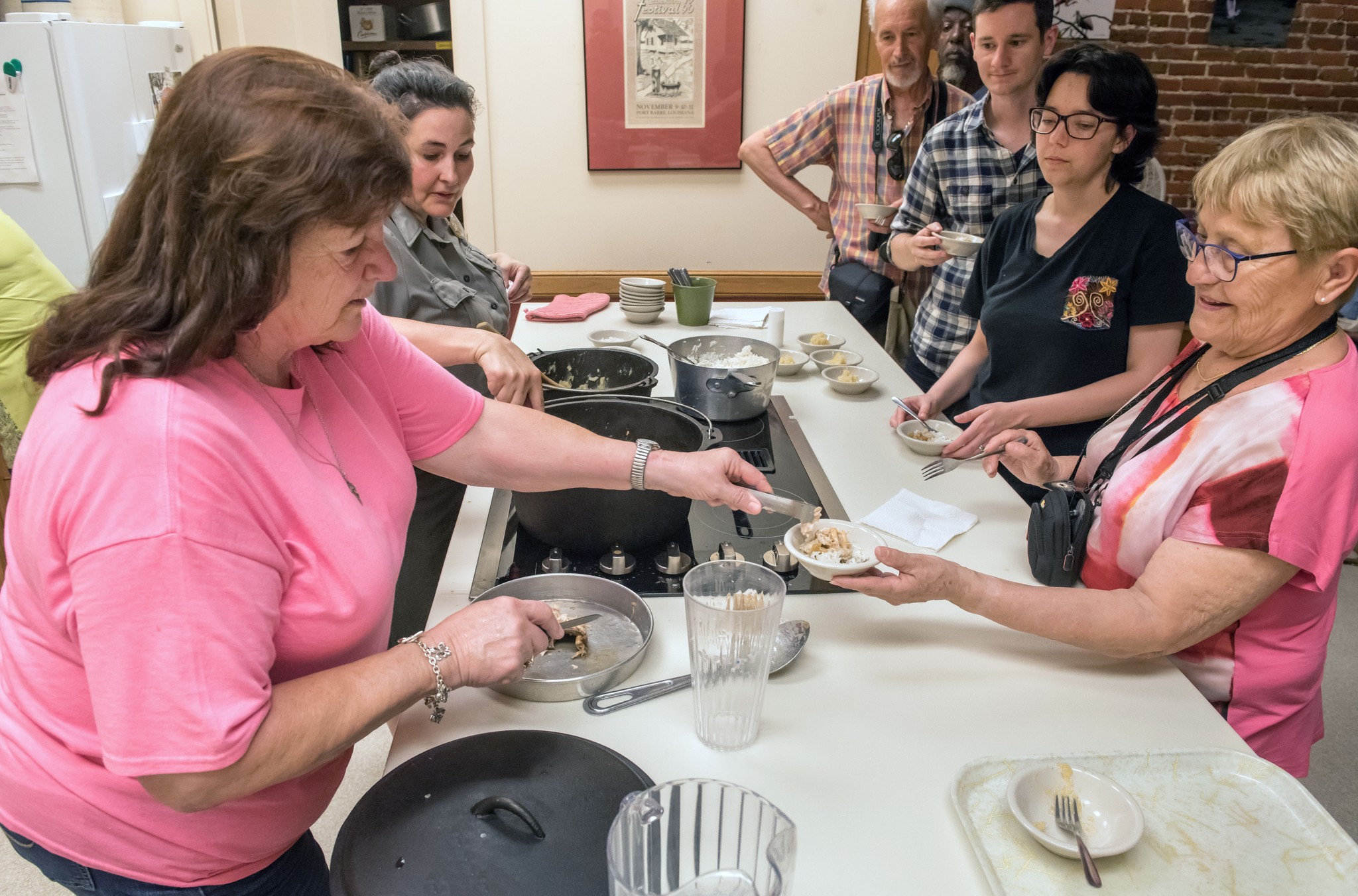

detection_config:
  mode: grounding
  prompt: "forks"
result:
[921,436,1028,481]
[875,215,886,226]
[1055,795,1102,888]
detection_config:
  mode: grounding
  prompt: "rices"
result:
[810,549,870,564]
[913,429,953,442]
[672,339,771,368]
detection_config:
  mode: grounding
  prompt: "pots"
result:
[397,1,451,39]
[526,347,659,401]
[511,393,723,556]
[667,335,781,421]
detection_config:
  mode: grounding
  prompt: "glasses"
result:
[886,131,905,182]
[1175,216,1313,282]
[1029,107,1120,140]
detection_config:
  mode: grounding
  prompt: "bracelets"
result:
[399,628,450,726]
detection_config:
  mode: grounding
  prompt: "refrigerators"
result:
[0,10,194,292]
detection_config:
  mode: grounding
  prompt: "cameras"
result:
[867,230,893,263]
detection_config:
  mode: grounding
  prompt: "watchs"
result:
[629,439,660,491]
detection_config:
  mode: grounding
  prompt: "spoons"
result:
[582,620,811,717]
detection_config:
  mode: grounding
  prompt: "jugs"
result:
[605,779,798,896]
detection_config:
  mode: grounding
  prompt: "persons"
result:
[828,118,1357,778]
[0,45,770,895]
[888,41,1197,506]
[0,210,83,582]
[364,48,542,736]
[932,2,990,102]
[735,0,977,369]
[879,0,1059,431]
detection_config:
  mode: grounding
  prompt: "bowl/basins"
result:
[783,518,889,582]
[1006,763,1145,860]
[619,277,667,324]
[598,346,641,355]
[776,349,810,376]
[821,365,880,395]
[587,329,639,348]
[855,203,898,220]
[809,349,864,371]
[896,419,964,456]
[796,332,846,355]
[931,231,985,257]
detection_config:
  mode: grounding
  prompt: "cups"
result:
[671,277,718,326]
[682,559,786,752]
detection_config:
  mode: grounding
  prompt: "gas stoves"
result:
[468,394,859,601]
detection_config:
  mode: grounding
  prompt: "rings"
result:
[979,445,985,454]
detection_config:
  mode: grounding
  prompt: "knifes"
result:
[539,614,603,633]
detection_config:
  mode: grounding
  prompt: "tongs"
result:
[733,483,817,523]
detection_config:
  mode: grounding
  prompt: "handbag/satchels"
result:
[828,262,903,328]
[1027,485,1093,588]
